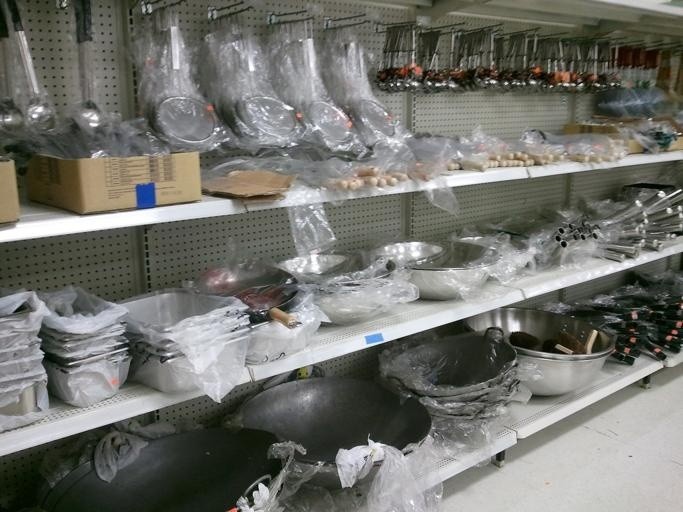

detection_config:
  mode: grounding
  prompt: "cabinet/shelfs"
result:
[0,1,682,512]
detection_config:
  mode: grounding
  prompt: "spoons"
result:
[370,25,623,95]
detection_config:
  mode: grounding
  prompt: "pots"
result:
[2,174,682,512]
